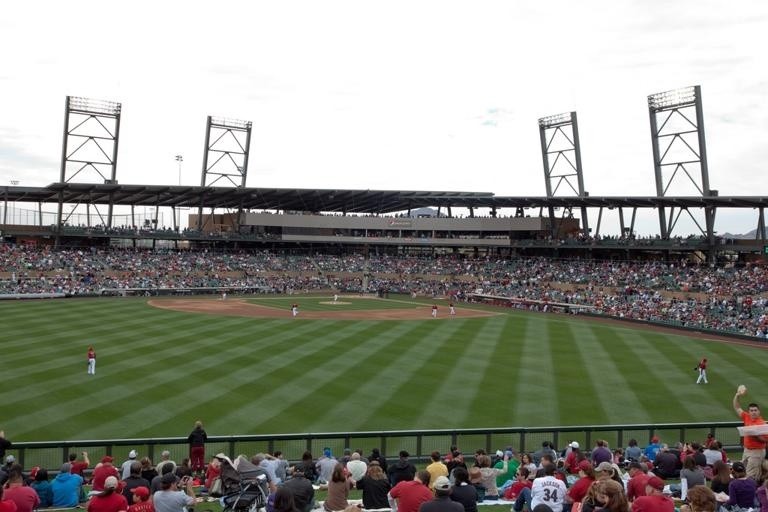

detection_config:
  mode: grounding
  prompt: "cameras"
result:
[502,455,512,460]
[184,477,189,482]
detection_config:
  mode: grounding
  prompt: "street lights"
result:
[175,155,183,227]
[9,178,20,225]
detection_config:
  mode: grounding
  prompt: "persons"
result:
[86,346,96,374]
[732,384,765,479]
[695,358,709,384]
[0,417,768,512]
[0,221,767,338]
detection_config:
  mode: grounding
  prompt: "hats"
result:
[11,435,742,495]
